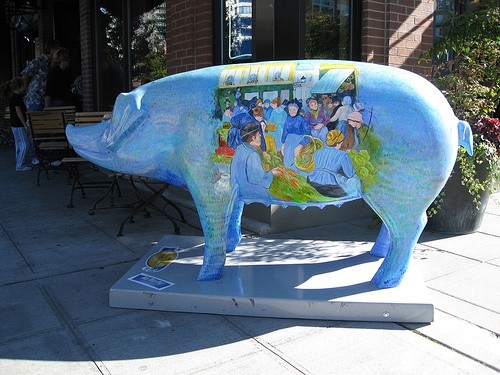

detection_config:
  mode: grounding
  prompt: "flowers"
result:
[21,53,48,78]
[416,0,500,218]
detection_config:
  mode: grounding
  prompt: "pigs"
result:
[65,60,474,291]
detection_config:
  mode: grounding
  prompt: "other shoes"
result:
[16,166,33,171]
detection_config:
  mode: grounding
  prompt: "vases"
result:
[25,78,45,110]
[425,155,495,233]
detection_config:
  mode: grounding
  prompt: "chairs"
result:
[0,106,186,237]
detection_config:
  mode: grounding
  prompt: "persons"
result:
[9,77,33,171]
[43,59,75,113]
[20,75,39,164]
